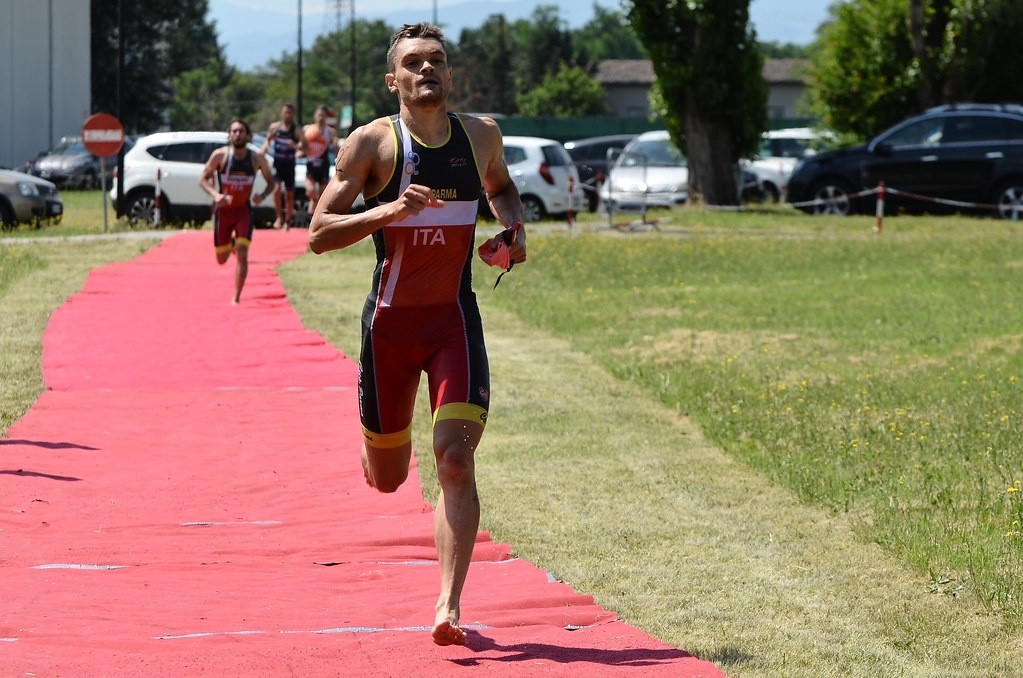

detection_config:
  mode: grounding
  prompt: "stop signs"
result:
[82,113,126,158]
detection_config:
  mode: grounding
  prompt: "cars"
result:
[562,134,639,212]
[737,127,840,209]
[255,126,348,168]
[31,132,134,191]
[477,133,589,224]
[0,168,64,231]
[783,103,1023,224]
[596,129,698,211]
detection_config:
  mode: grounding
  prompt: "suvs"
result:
[109,129,366,233]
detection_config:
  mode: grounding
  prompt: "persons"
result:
[260,103,343,230]
[308,22,528,647]
[199,118,276,305]
